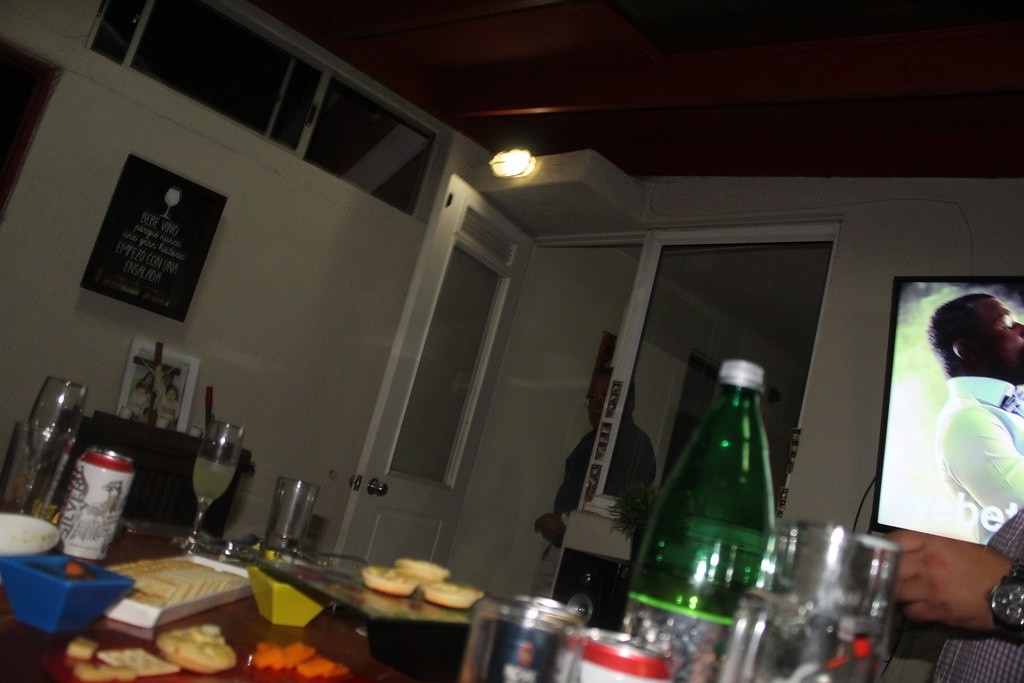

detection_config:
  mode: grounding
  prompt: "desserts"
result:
[364,558,484,609]
[66,636,181,682]
[156,625,236,673]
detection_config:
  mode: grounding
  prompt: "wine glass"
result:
[160,189,180,220]
[171,420,246,553]
[17,377,89,514]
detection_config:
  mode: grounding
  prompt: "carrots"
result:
[253,640,349,677]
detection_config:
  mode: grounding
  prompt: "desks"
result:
[249,557,474,683]
[0,521,421,683]
[32,409,255,541]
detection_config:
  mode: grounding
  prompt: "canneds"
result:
[57,448,134,563]
[550,626,671,683]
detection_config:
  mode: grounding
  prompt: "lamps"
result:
[488,147,536,179]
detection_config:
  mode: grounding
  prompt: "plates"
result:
[0,514,60,556]
[101,555,255,627]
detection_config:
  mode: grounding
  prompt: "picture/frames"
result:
[115,336,201,434]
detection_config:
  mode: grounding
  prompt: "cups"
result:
[627,528,902,683]
[257,477,319,566]
[0,423,66,514]
[119,406,134,419]
[190,426,202,438]
[155,417,169,429]
[460,596,584,683]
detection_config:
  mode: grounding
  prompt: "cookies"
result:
[104,560,251,608]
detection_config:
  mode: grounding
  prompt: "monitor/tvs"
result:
[869,276,1024,536]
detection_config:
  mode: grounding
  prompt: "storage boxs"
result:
[0,554,325,636]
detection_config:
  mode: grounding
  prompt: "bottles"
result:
[622,359,776,683]
